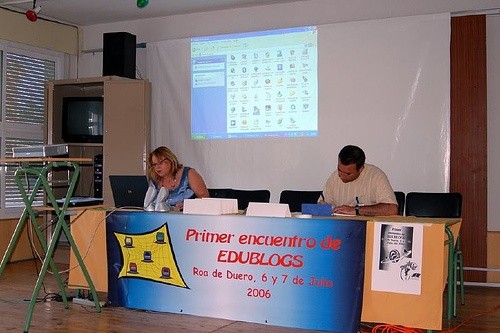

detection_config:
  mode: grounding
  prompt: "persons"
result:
[144,146,210,211]
[317,145,399,215]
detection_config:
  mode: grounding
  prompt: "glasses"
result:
[151,159,169,167]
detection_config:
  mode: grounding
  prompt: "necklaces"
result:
[171,179,176,187]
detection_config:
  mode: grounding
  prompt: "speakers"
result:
[102,32,136,78]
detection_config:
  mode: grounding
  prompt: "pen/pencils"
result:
[320,192,325,202]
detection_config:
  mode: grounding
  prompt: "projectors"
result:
[12,144,69,158]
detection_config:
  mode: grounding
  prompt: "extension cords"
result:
[72,297,106,307]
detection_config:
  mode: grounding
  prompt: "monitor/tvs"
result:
[62,97,103,144]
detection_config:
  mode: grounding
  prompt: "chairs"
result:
[207,188,465,317]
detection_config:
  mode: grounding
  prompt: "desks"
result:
[0,157,101,333]
[68,206,464,333]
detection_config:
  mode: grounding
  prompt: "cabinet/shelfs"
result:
[44,76,153,264]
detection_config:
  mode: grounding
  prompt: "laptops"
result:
[109,175,148,209]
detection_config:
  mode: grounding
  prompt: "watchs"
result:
[355,205,359,215]
